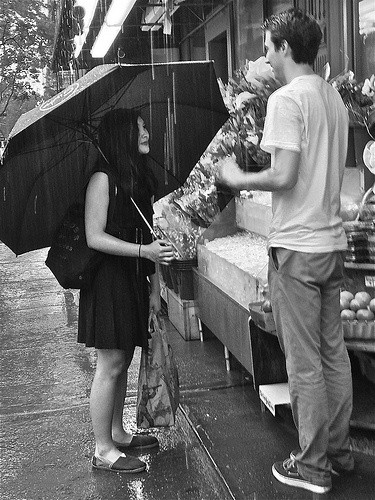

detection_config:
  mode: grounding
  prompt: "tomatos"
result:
[262,300,272,313]
[337,290,375,320]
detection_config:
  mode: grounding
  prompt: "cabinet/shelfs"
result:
[192,120,375,439]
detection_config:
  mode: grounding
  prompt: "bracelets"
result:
[139,243,141,257]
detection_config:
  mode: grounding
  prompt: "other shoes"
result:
[116,435,160,451]
[90,451,146,473]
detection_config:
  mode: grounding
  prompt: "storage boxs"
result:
[168,288,212,341]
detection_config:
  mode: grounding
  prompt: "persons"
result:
[215,8,360,493]
[74,108,178,475]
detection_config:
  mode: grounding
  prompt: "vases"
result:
[158,255,194,300]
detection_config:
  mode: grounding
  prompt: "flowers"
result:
[158,56,375,265]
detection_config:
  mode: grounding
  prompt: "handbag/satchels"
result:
[135,312,180,430]
[44,165,117,291]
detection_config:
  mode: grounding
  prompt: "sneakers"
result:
[271,450,333,494]
[332,454,355,472]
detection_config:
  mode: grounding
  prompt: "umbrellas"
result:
[0,60,231,257]
